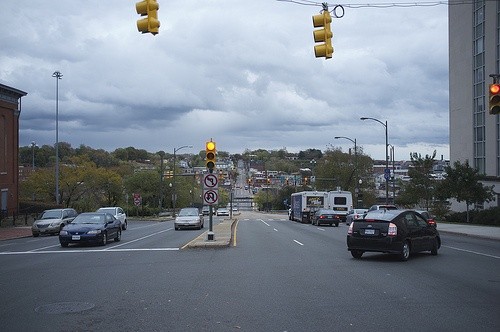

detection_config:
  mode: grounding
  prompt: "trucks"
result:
[288,190,353,224]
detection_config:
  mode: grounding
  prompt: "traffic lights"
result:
[312,11,334,59]
[487,83,500,115]
[206,142,216,169]
[136,0,160,36]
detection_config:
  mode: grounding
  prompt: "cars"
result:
[311,209,340,227]
[347,209,441,262]
[174,208,204,231]
[405,211,437,229]
[201,205,238,217]
[344,208,368,225]
[94,207,128,230]
[32,208,79,238]
[59,213,122,248]
[364,205,399,214]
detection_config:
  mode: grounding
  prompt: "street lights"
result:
[51,70,63,208]
[334,136,358,208]
[173,145,192,218]
[31,141,36,200]
[360,117,388,205]
[387,144,395,204]
